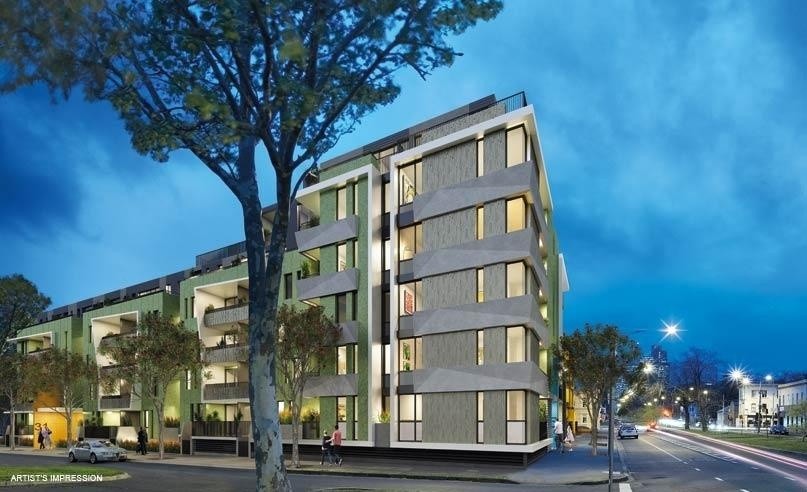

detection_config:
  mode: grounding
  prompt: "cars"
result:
[68,440,127,464]
[617,424,639,440]
[770,424,789,435]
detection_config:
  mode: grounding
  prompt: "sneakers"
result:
[320,459,343,467]
[568,444,572,451]
